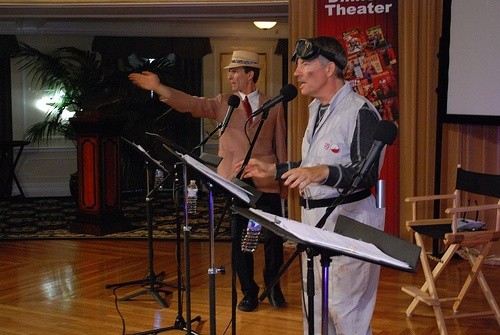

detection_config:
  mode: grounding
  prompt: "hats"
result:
[224,50,262,69]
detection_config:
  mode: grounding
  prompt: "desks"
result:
[0,141,31,196]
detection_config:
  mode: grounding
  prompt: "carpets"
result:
[0,196,265,243]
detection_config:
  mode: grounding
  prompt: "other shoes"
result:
[267,288,285,306]
[238,296,258,312]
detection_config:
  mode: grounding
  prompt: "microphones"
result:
[252,83,298,116]
[352,120,397,188]
[220,95,240,134]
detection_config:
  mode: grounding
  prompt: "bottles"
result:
[186,180,198,216]
[241,218,262,252]
[154,167,165,189]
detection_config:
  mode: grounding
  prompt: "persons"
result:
[234,35,387,335]
[128,50,288,311]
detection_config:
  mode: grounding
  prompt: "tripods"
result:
[106,133,204,335]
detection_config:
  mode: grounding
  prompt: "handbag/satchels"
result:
[450,199,486,259]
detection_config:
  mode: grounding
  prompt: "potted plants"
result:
[9,40,176,215]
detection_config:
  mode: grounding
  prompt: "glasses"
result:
[290,39,345,71]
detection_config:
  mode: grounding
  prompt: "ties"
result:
[242,95,253,125]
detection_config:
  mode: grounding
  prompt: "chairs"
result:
[402,164,500,335]
